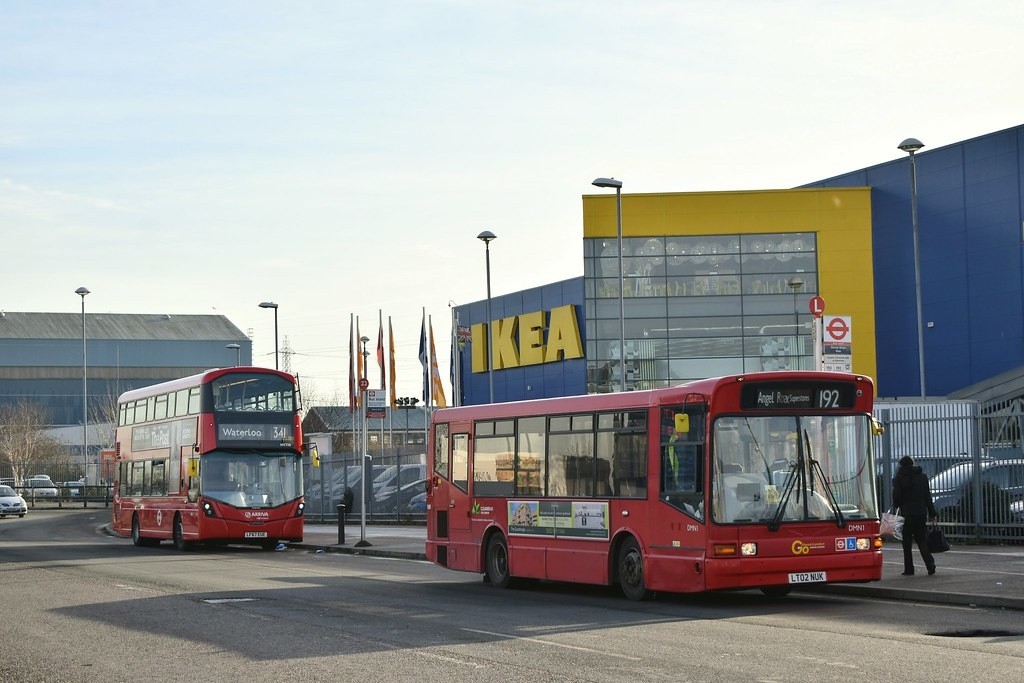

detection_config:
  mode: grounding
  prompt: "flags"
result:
[349,307,456,414]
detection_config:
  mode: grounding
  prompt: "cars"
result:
[0,485,28,518]
[0,474,67,498]
[305,463,427,519]
[23,478,58,501]
[60,481,86,501]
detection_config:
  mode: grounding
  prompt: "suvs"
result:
[927,458,1024,546]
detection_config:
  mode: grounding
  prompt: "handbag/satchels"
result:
[928,520,950,552]
[880,507,905,541]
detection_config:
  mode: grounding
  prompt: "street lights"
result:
[225,343,241,366]
[74,287,92,509]
[591,177,625,391]
[394,396,419,446]
[258,301,279,370]
[477,230,497,404]
[897,138,928,401]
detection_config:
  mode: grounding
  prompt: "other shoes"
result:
[901,572,914,575]
[928,564,936,575]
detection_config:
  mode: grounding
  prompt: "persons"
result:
[889,456,937,575]
[343,487,354,513]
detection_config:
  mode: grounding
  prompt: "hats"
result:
[899,456,913,466]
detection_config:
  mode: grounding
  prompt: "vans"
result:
[875,454,996,516]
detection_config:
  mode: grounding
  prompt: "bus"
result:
[424,370,885,604]
[110,365,320,552]
